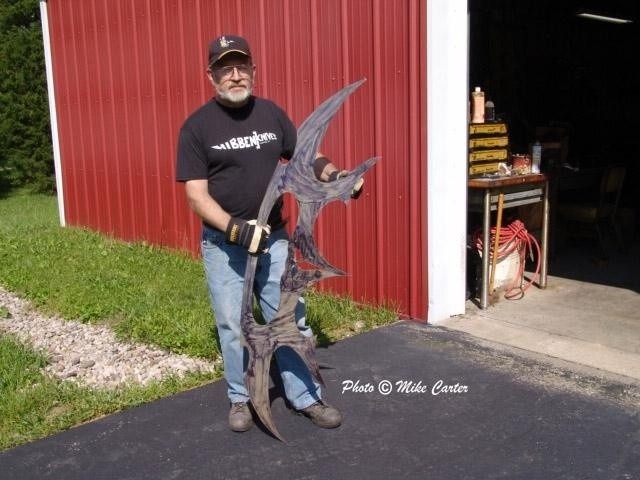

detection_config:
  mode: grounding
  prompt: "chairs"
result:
[558,161,628,261]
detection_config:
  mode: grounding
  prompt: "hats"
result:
[205,33,252,69]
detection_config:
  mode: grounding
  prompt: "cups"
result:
[513,153,530,172]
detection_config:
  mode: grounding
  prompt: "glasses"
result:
[216,62,252,81]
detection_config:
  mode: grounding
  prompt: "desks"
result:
[466,173,552,309]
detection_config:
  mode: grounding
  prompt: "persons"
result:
[175,36,366,432]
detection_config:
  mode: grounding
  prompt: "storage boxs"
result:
[468,239,527,293]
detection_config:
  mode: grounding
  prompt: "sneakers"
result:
[227,401,254,434]
[290,395,343,431]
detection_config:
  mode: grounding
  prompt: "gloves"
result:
[223,216,275,257]
[326,168,365,200]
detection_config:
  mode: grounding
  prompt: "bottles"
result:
[484,101,496,121]
[530,141,542,174]
[472,86,484,125]
